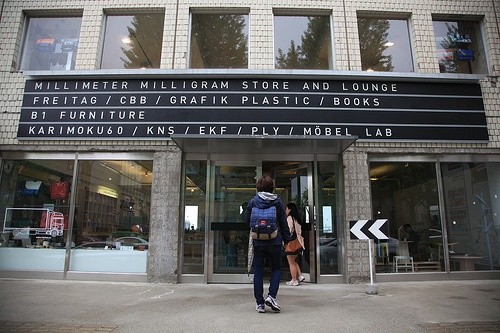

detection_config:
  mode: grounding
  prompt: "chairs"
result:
[392,241,414,273]
[435,242,458,272]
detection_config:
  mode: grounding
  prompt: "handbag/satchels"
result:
[284,230,306,254]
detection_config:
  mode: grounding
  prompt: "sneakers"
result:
[255,303,265,313]
[264,295,281,311]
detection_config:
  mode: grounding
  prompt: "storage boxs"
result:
[437,49,454,60]
[456,50,474,60]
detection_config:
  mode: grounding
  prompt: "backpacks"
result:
[250,206,279,241]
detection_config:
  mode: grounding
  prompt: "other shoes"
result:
[298,275,305,283]
[286,279,298,286]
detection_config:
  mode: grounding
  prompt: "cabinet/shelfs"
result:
[437,41,475,74]
[77,190,118,236]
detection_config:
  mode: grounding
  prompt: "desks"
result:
[185,241,205,265]
[451,256,485,271]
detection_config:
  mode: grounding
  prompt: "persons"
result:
[402,223,425,262]
[284,202,305,285]
[243,176,291,313]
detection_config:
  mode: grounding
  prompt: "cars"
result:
[114,237,148,250]
[72,241,116,250]
